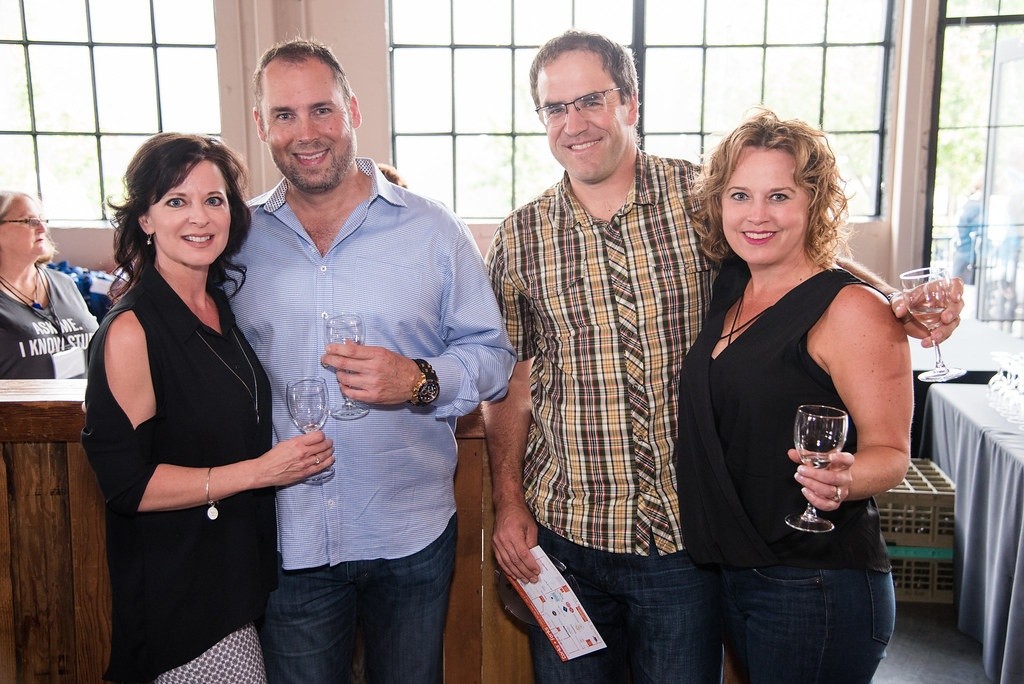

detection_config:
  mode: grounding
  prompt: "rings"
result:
[314,453,320,465]
[833,486,841,503]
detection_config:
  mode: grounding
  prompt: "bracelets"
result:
[206,466,219,520]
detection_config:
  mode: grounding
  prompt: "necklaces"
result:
[0,269,46,310]
[199,327,260,424]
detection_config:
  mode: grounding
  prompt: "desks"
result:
[911,316,1024,461]
[923,381,1024,684]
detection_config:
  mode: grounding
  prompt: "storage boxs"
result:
[877,459,958,548]
[886,547,955,605]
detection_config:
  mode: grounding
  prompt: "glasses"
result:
[539,89,623,127]
[0,219,49,229]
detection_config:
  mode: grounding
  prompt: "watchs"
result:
[406,359,441,408]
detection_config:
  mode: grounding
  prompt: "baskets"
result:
[875,457,956,604]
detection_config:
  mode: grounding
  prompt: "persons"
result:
[478,30,964,684]
[0,192,100,378]
[673,106,914,684]
[218,36,517,683]
[80,131,336,684]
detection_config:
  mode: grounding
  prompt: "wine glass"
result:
[899,266,966,382]
[784,403,849,532]
[324,308,370,421]
[985,349,1024,429]
[286,376,335,481]
